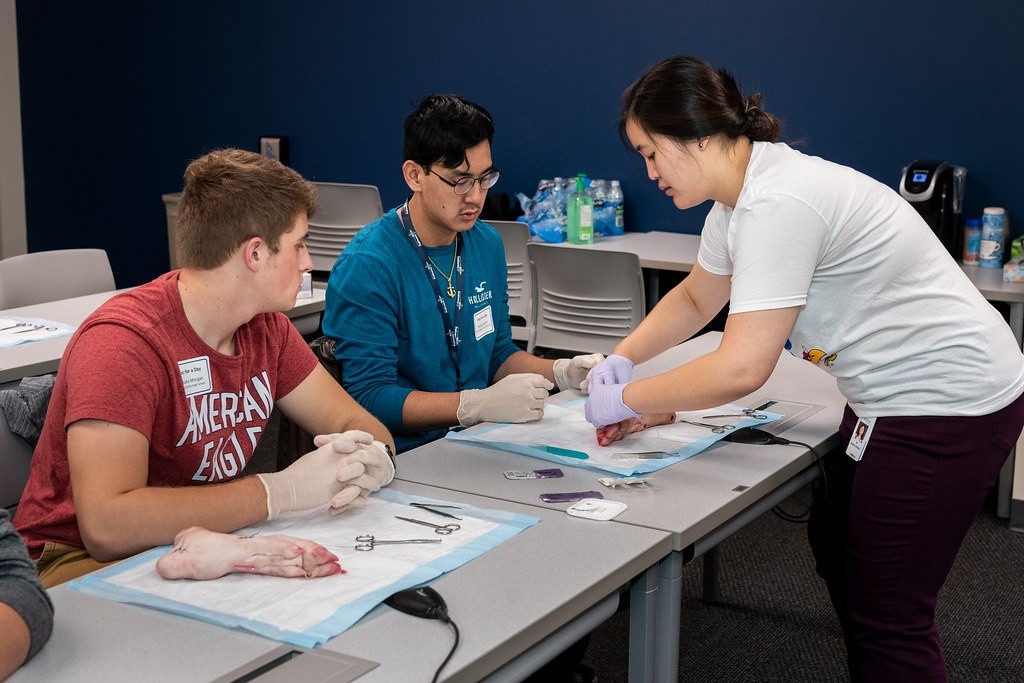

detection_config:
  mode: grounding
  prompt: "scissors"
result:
[395,516,461,535]
[355,535,442,550]
[702,408,768,420]
[0,322,26,331]
[680,420,736,434]
[13,324,58,333]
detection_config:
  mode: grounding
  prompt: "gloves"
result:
[587,354,634,396]
[584,381,644,436]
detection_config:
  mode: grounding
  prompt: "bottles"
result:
[980,208,1005,269]
[963,218,983,265]
[534,178,624,236]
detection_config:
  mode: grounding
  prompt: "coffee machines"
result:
[898,160,967,263]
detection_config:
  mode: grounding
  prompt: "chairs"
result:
[526,243,645,358]
[0,248,116,312]
[305,182,383,290]
[481,220,531,341]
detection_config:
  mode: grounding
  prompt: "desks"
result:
[0,231,1024,683]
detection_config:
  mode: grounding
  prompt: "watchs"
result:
[374,441,398,469]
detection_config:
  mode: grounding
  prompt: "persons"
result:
[11,147,395,586]
[578,55,1024,683]
[0,512,55,683]
[855,425,865,441]
[321,94,604,456]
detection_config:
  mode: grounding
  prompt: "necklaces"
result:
[414,235,459,297]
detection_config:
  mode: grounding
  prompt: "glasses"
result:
[423,166,501,195]
